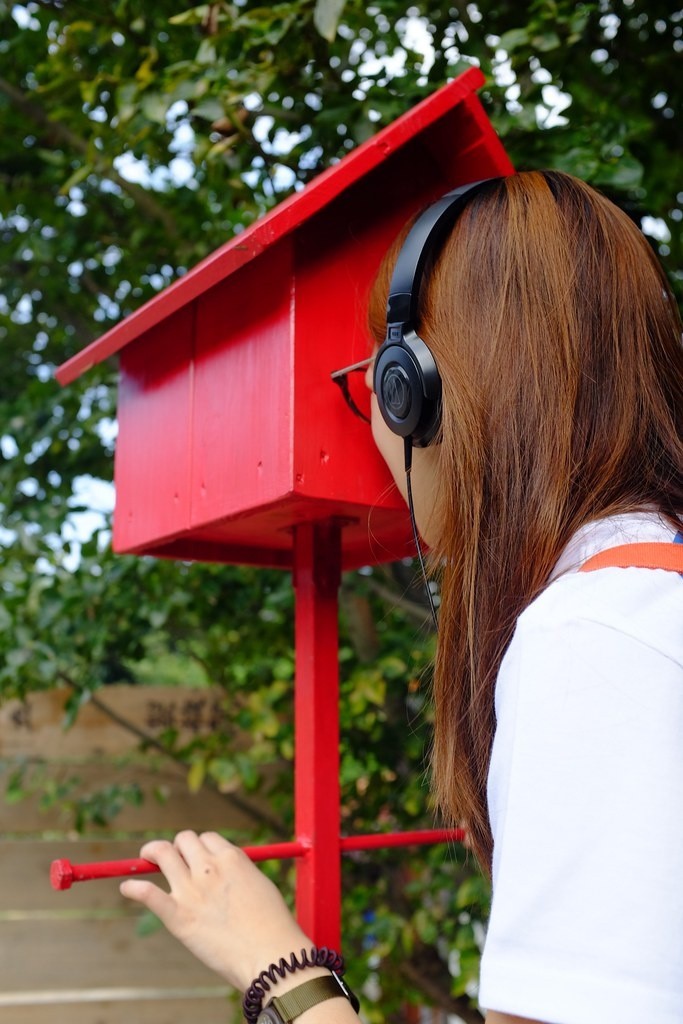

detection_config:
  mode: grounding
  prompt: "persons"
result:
[119,169,683,1024]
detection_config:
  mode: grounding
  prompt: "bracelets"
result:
[242,947,346,1024]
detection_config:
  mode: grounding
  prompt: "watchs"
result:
[257,971,359,1024]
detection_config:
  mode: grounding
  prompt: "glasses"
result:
[330,355,377,424]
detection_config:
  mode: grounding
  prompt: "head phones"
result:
[373,175,503,450]
